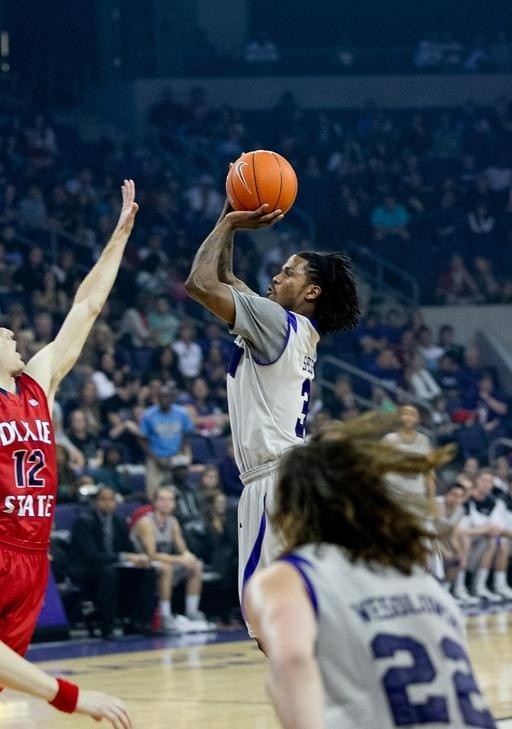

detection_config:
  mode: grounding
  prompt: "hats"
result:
[147,226,167,240]
[48,202,70,222]
[124,370,142,380]
[234,234,253,250]
[122,283,144,308]
[100,394,131,423]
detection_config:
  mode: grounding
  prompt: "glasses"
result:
[201,183,211,188]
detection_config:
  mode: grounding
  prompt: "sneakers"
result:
[494,585,512,600]
[471,587,502,603]
[186,612,216,631]
[151,615,182,635]
[454,589,480,605]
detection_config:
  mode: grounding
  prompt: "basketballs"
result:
[225,150,299,220]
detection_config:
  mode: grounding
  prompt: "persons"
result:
[493,456,512,512]
[380,405,435,527]
[242,407,499,729]
[202,491,246,626]
[128,488,216,633]
[0,178,139,693]
[159,453,213,564]
[314,307,511,444]
[0,226,114,366]
[423,483,481,606]
[0,24,511,246]
[57,230,240,503]
[463,467,512,602]
[68,487,150,642]
[183,152,363,660]
[0,639,133,729]
[463,456,479,475]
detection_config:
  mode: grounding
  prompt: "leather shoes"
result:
[129,622,153,635]
[101,627,121,641]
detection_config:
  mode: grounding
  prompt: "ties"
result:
[103,520,112,551]
